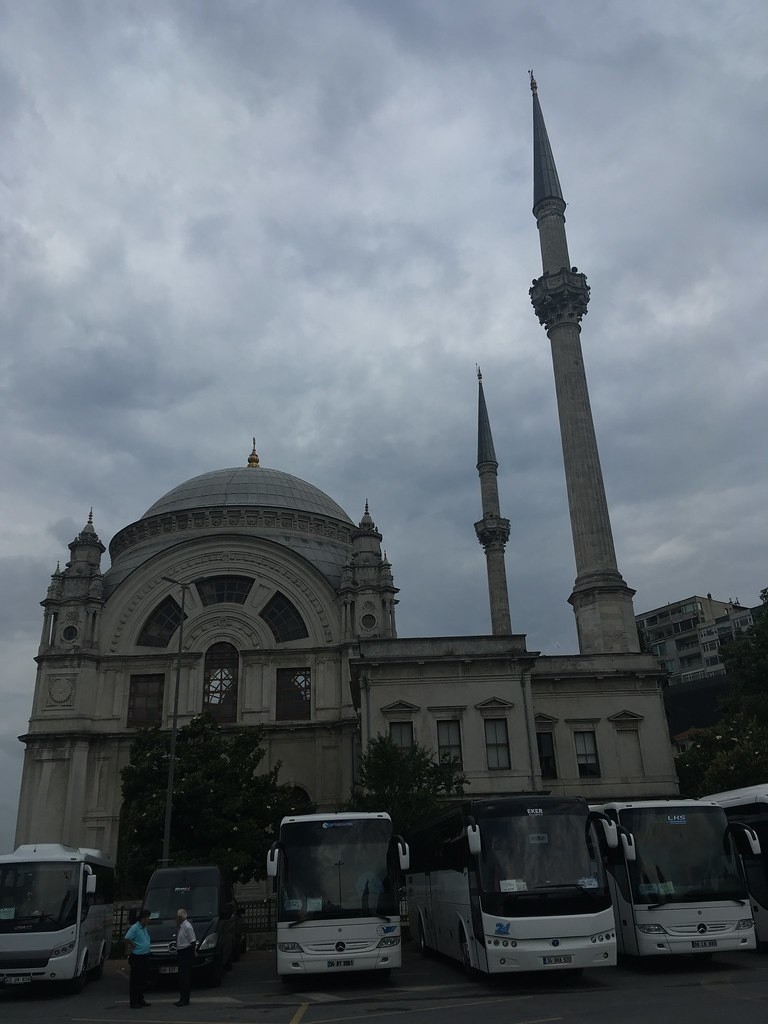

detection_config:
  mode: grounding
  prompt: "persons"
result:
[173,909,196,1006]
[124,909,152,1009]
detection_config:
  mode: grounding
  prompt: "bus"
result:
[696,784,768,942]
[266,809,409,980]
[593,799,762,962]
[399,794,619,981]
[0,843,115,992]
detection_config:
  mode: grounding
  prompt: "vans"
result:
[128,864,243,991]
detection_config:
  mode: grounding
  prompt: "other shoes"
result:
[174,1001,190,1006]
[130,1001,151,1009]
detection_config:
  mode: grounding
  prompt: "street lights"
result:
[159,576,206,870]
